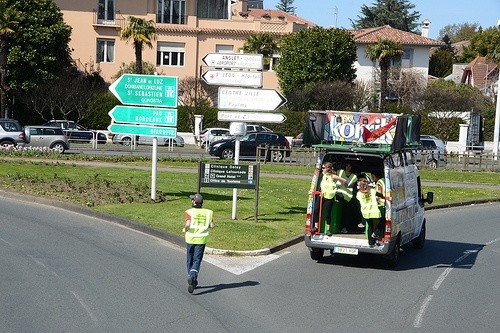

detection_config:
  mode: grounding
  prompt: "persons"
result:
[183,194,215,293]
[319,161,386,247]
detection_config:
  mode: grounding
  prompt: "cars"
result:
[112,134,185,147]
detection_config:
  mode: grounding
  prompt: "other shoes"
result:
[327,230,332,236]
[309,227,318,232]
[187,278,197,293]
[371,232,382,239]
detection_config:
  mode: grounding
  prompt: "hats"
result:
[190,194,203,204]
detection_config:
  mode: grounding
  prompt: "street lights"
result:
[477,62,488,95]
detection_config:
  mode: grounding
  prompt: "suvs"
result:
[41,120,107,144]
[417,135,447,168]
[0,118,26,147]
[17,126,70,154]
[246,125,274,132]
[196,127,230,148]
[208,131,291,162]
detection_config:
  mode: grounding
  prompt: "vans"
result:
[304,143,435,260]
